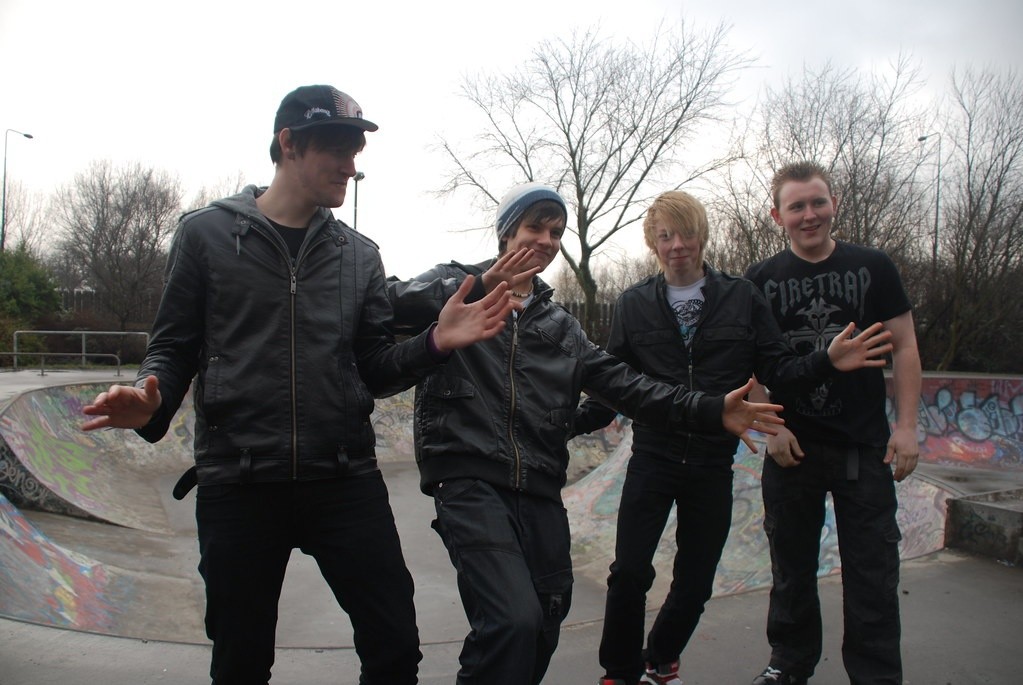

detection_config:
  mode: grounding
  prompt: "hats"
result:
[495,182,567,241]
[274,84,379,133]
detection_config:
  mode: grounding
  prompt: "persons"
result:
[384,186,786,685]
[739,159,924,684]
[81,85,515,685]
[567,186,893,685]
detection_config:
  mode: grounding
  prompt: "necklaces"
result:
[512,283,535,298]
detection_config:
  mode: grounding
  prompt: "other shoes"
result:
[750,664,808,685]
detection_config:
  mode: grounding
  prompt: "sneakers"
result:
[597,656,685,685]
